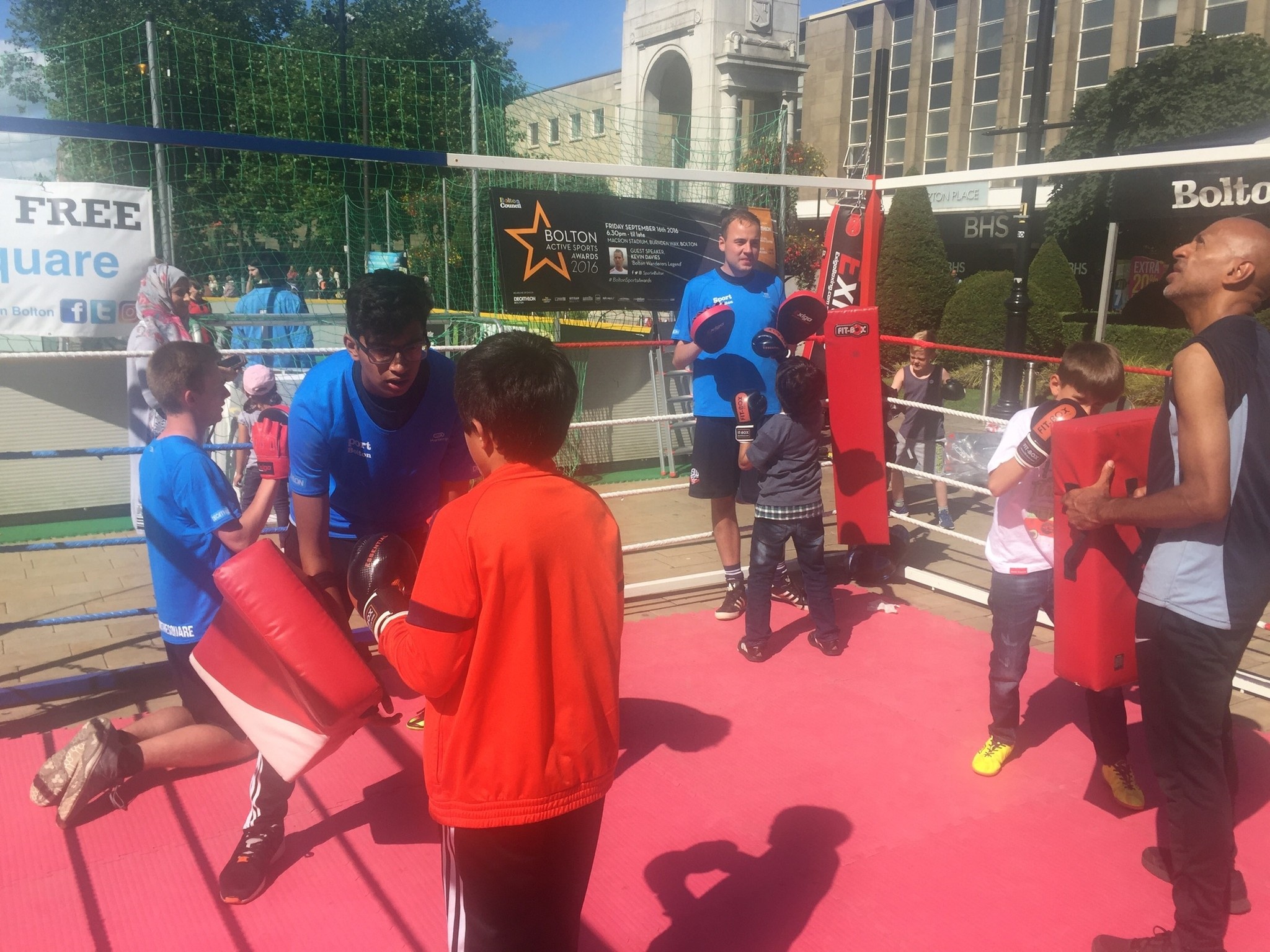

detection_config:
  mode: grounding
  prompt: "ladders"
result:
[648,310,696,477]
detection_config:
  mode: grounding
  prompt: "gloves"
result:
[731,389,768,442]
[1015,398,1088,468]
[942,378,965,401]
[347,531,418,645]
[752,328,791,362]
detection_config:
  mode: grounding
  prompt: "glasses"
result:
[354,328,431,364]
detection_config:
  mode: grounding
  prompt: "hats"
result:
[244,364,276,395]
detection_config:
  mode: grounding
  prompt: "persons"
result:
[1059,218,1270,952]
[32,208,1123,952]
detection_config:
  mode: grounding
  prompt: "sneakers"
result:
[1092,925,1225,952]
[1139,846,1251,914]
[807,631,842,656]
[889,501,909,516]
[972,736,1015,777]
[1102,759,1145,811]
[737,636,766,662]
[219,823,284,904]
[770,574,809,611]
[715,577,747,620]
[938,509,954,530]
[29,716,123,831]
